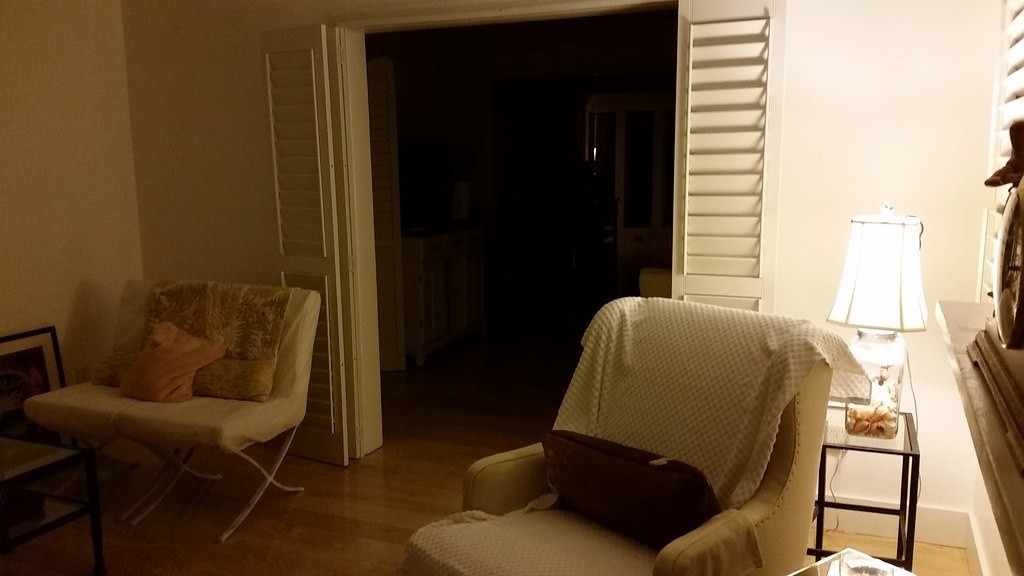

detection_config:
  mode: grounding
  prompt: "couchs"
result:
[399,296,873,576]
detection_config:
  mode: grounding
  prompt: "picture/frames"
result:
[0,325,79,478]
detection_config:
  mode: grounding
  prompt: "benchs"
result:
[22,277,322,546]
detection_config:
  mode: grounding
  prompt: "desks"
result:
[787,547,918,576]
[814,403,921,573]
[0,435,109,576]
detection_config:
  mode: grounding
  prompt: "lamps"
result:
[825,213,930,441]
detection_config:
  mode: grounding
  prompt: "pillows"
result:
[117,321,227,403]
[538,430,723,552]
[90,278,293,402]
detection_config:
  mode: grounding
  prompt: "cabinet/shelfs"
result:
[401,215,490,365]
